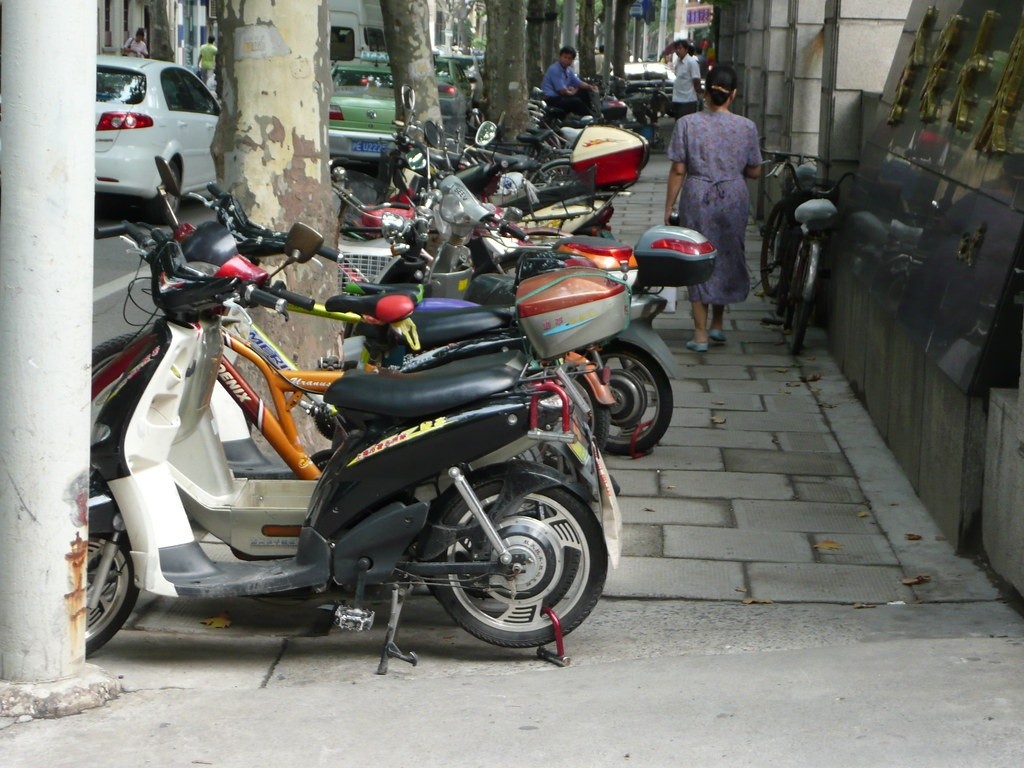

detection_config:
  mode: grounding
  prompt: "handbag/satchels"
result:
[669,208,680,226]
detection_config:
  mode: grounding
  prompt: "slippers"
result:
[708,328,727,341]
[686,340,708,352]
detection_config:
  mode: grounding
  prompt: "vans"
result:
[325,1,388,64]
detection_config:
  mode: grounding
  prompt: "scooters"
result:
[85,86,719,679]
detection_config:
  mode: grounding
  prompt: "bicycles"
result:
[758,136,862,357]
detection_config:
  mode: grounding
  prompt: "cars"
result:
[611,61,677,109]
[327,50,400,161]
[434,59,477,118]
[477,54,485,77]
[436,54,484,102]
[95,55,222,225]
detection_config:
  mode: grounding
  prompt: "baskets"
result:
[338,253,392,295]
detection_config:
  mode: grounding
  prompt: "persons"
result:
[665,65,762,350]
[661,40,717,120]
[540,44,603,120]
[197,35,219,89]
[594,44,613,74]
[121,28,149,57]
